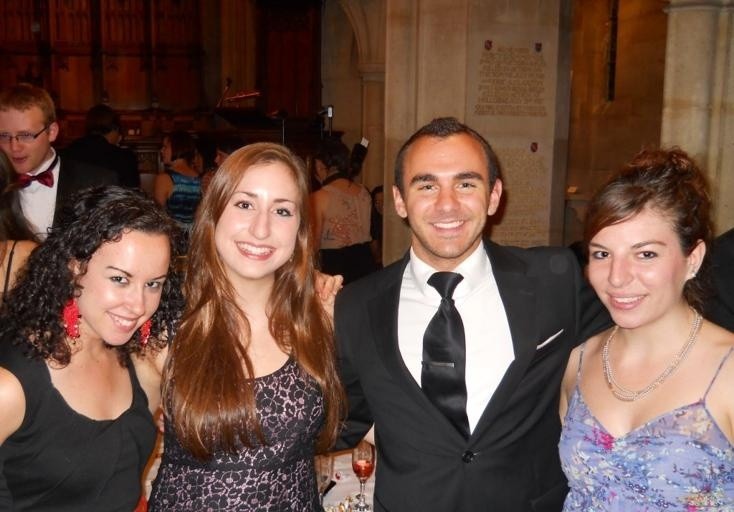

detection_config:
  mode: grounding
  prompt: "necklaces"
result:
[601,307,702,402]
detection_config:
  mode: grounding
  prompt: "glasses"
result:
[0,124,49,145]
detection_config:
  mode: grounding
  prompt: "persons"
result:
[704,229,733,330]
[314,116,610,511]
[558,141,733,511]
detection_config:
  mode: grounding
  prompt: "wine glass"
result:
[313,455,334,504]
[351,441,376,509]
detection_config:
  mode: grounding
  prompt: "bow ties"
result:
[14,154,59,189]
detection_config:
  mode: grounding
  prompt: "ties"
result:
[416,268,472,442]
